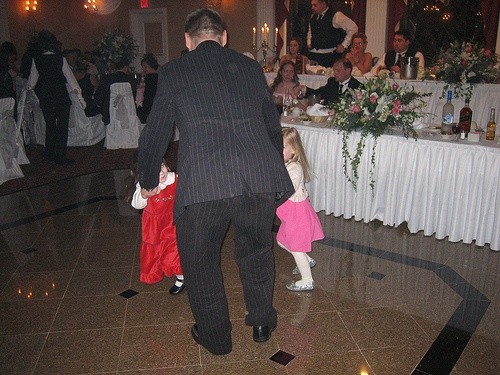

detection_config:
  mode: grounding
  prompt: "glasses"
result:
[353,41,364,46]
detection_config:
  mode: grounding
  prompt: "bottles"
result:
[486,108,496,140]
[441,91,454,135]
[284,88,291,113]
[459,99,473,137]
[297,90,303,100]
[292,102,300,118]
[284,108,287,116]
[460,130,465,140]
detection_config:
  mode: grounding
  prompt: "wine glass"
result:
[451,123,461,142]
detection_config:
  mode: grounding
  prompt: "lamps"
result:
[84,0,99,15]
[26,1,41,17]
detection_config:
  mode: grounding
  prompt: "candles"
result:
[261,23,269,44]
[274,27,278,46]
[252,27,256,45]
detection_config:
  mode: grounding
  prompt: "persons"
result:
[335,32,372,77]
[56,40,160,124]
[25,30,87,165]
[279,37,308,74]
[276,127,324,292]
[370,30,424,79]
[291,57,365,110]
[306,0,358,68]
[0,41,26,146]
[270,60,308,113]
[130,158,187,295]
[138,8,295,355]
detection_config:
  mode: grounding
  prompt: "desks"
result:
[285,111,500,253]
[264,69,500,133]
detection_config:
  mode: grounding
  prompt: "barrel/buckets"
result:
[400,57,419,80]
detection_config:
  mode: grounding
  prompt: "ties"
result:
[338,81,349,94]
[315,15,323,51]
[394,54,402,67]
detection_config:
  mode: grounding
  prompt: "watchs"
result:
[145,187,156,192]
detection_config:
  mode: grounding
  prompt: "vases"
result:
[464,63,483,84]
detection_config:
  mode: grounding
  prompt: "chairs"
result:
[0,75,143,184]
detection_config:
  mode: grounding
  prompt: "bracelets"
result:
[355,60,360,66]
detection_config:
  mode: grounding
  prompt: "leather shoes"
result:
[189,323,232,355]
[253,307,277,342]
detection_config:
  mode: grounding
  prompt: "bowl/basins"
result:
[310,116,329,123]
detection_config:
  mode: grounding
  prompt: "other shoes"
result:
[169,278,186,295]
[292,258,317,275]
[41,150,55,156]
[55,152,66,159]
[286,280,316,291]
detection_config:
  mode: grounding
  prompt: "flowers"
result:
[430,41,500,103]
[335,76,426,190]
[98,27,140,68]
